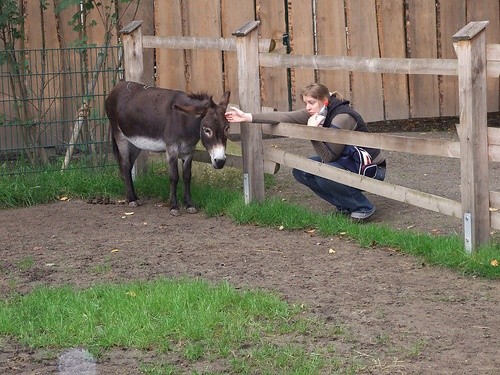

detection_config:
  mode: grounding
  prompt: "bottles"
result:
[317,100,329,128]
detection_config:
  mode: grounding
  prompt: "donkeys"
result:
[105,81,231,217]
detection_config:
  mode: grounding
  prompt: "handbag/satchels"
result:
[336,146,385,180]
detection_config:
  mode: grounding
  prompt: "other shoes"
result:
[351,206,378,219]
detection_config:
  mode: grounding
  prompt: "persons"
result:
[225,83,386,218]
